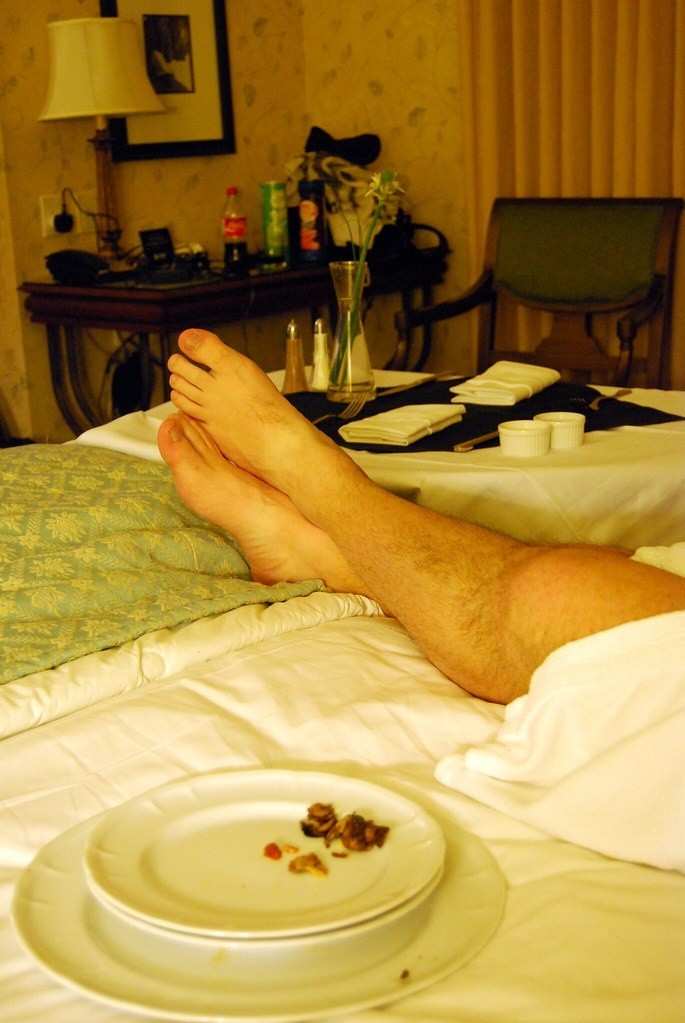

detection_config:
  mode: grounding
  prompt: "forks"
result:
[312,393,369,425]
[569,389,633,409]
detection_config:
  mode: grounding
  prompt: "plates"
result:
[13,805,508,1023]
[85,770,446,950]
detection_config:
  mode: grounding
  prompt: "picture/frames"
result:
[99,0,237,165]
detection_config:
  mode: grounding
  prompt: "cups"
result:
[258,233,287,272]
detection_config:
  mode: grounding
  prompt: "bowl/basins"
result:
[533,412,585,450]
[498,420,551,457]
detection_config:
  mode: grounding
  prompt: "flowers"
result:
[331,167,407,393]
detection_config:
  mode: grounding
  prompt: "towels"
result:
[337,402,467,447]
[448,359,562,407]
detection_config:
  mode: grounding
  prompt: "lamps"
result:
[37,15,167,258]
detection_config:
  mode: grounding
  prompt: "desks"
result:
[15,222,454,436]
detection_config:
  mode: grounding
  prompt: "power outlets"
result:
[40,190,66,236]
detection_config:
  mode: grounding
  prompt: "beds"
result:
[73,363,685,547]
[0,443,685,1023]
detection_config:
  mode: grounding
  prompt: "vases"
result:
[327,261,377,402]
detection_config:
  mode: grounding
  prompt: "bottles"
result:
[222,187,251,274]
[311,319,335,393]
[282,320,309,395]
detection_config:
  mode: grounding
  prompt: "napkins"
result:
[338,402,466,446]
[449,360,561,406]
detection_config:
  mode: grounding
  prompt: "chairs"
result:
[386,198,685,391]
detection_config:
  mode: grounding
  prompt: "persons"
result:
[155,327,685,877]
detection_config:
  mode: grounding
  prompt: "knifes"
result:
[377,370,450,396]
